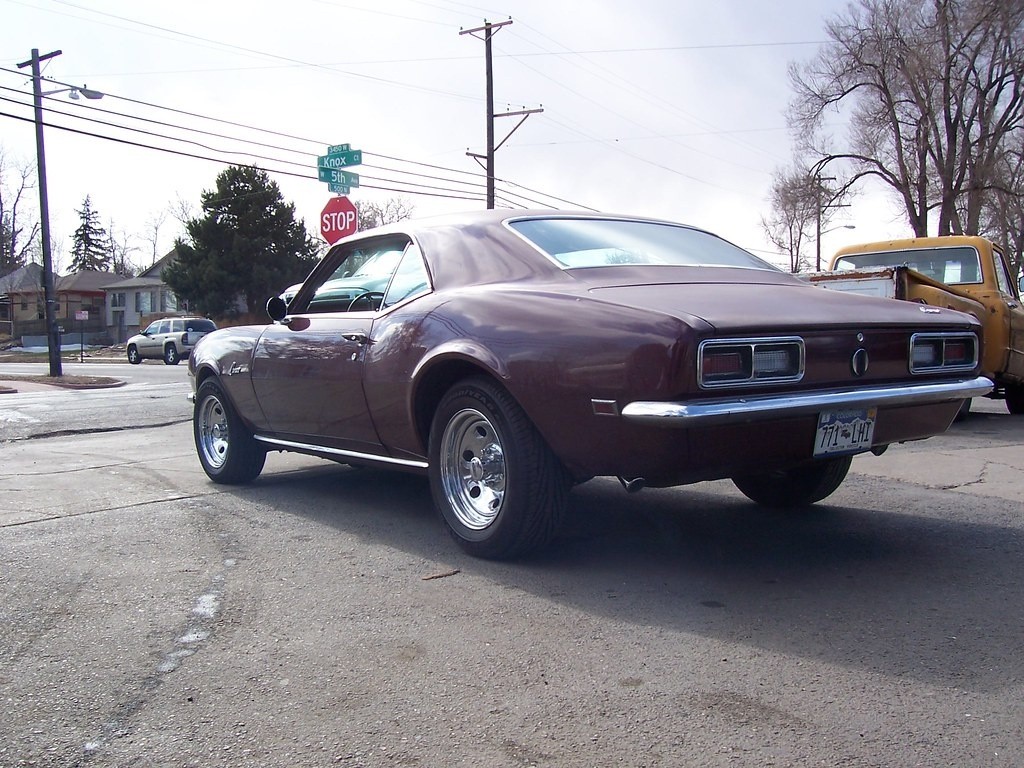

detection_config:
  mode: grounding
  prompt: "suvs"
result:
[126,316,218,365]
[274,248,428,312]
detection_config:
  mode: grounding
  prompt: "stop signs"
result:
[318,198,357,247]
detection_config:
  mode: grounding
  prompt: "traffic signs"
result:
[316,143,362,195]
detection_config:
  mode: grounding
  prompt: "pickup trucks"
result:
[797,233,1024,422]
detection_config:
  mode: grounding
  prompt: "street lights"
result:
[34,85,105,373]
[816,225,855,271]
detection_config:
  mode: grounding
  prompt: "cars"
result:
[188,206,994,556]
